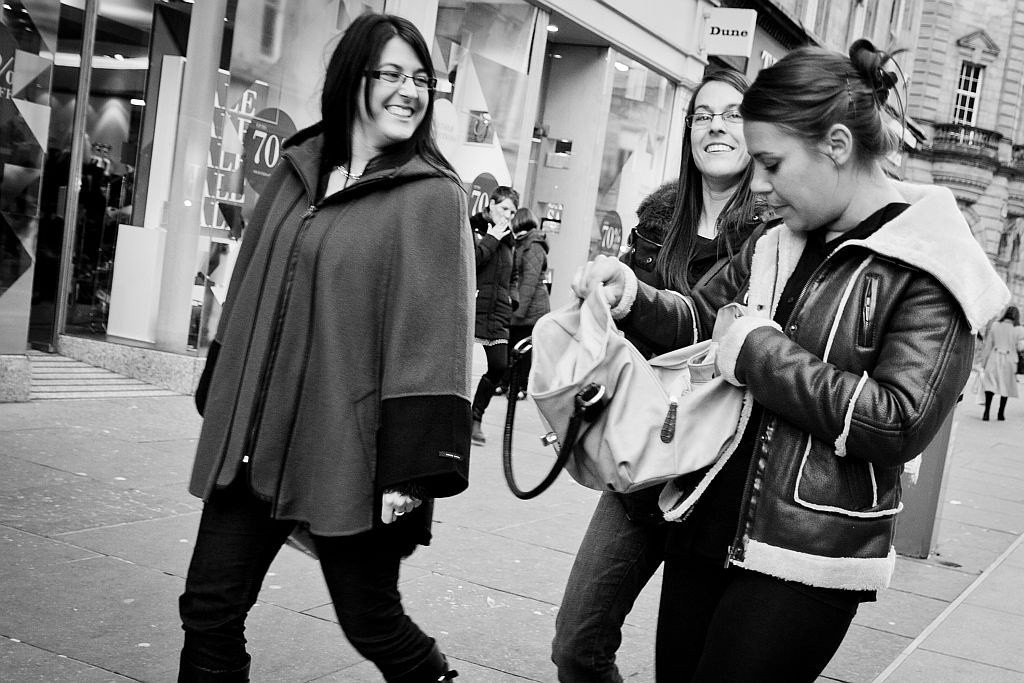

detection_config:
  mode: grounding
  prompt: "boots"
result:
[177,648,251,683]
[383,637,459,683]
[998,395,1007,420]
[983,391,994,421]
[472,375,499,443]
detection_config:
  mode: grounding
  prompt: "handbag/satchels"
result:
[504,282,763,500]
[1015,351,1024,374]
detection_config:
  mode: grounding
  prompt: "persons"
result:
[551,72,776,683]
[472,186,520,445]
[976,305,1024,423]
[178,15,476,683]
[493,206,550,399]
[573,37,1013,683]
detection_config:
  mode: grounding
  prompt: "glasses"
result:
[362,70,437,88]
[685,111,744,129]
